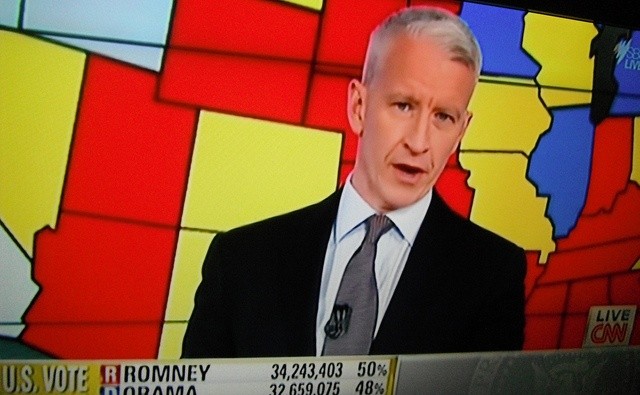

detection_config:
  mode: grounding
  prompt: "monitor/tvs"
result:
[0,0,639,394]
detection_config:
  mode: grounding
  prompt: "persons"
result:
[179,8,527,359]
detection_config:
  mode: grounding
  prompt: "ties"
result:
[320,216,394,356]
